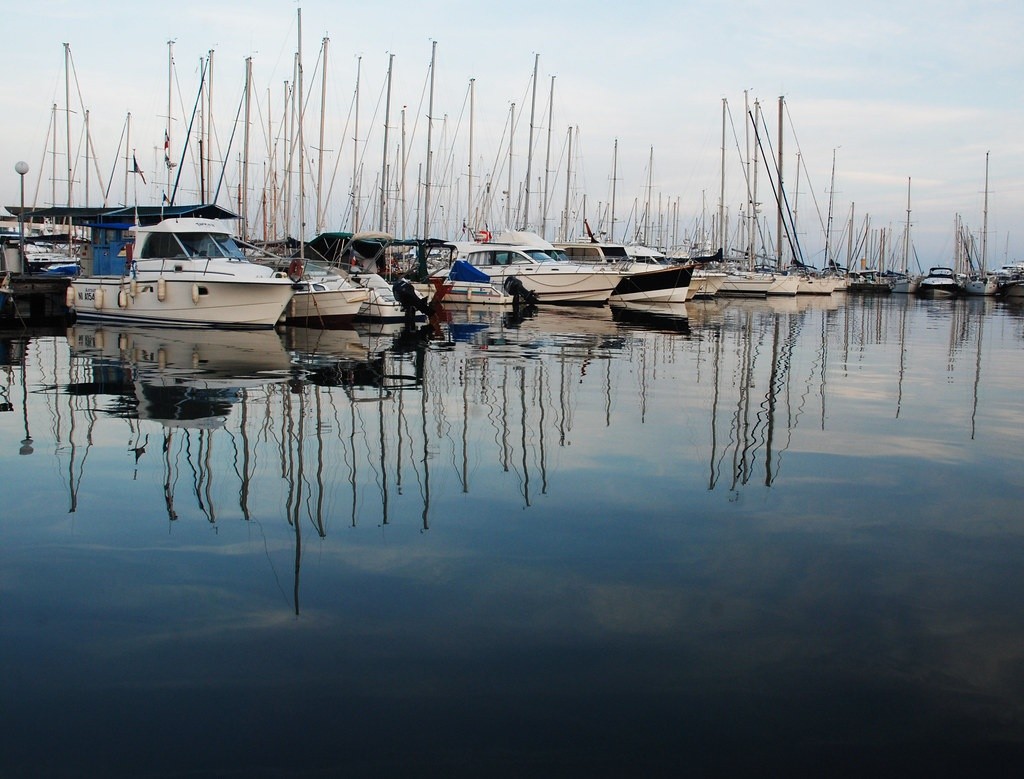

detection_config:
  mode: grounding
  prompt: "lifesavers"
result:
[288,259,303,280]
[385,259,399,275]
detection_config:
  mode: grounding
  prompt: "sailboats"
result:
[23,6,1024,331]
[3,296,1021,627]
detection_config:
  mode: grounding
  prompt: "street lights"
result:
[15,162,29,257]
